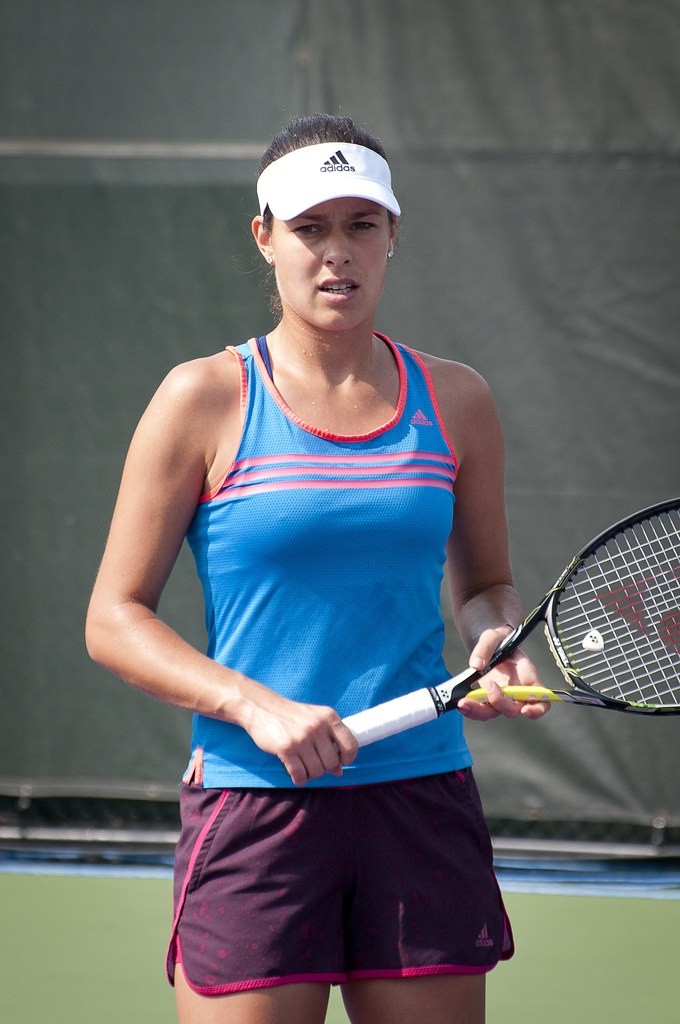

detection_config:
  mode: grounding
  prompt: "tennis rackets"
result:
[329,494,680,757]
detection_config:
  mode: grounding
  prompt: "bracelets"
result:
[505,622,516,631]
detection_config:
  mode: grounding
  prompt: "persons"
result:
[82,112,552,1024]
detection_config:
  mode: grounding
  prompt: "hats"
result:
[256,142,401,221]
